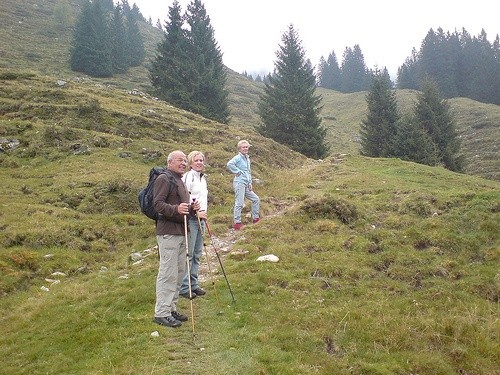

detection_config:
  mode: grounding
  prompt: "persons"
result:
[227,140,259,229]
[154,150,200,327]
[179,150,208,299]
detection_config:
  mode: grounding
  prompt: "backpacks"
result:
[138,167,174,220]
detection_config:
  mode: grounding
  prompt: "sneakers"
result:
[253,218,259,224]
[180,291,197,298]
[193,288,206,295]
[233,223,242,231]
[171,311,188,321]
[155,316,181,327]
